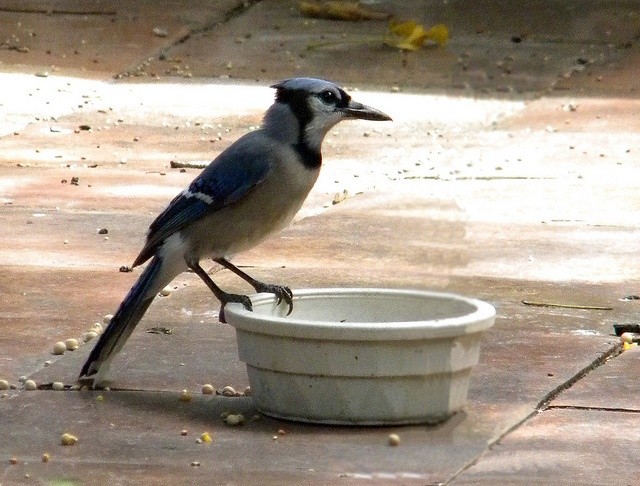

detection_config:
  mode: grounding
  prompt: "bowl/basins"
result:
[224,287,497,428]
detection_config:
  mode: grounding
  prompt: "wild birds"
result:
[77,76,394,394]
